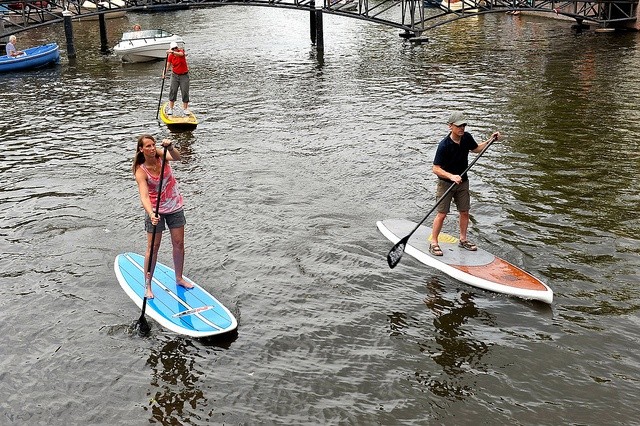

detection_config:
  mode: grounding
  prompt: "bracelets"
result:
[145,210,156,220]
[171,51,174,54]
[168,147,174,152]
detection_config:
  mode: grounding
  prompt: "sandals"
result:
[428,243,443,256]
[458,239,477,251]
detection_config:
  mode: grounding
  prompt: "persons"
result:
[131,135,194,300]
[162,42,191,115]
[429,112,502,257]
[6,35,24,57]
[133,24,141,31]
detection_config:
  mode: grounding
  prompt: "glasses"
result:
[171,46,178,49]
[454,123,467,127]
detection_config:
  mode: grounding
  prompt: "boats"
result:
[0,42,59,72]
[113,29,186,63]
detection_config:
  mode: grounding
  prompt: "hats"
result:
[448,112,469,125]
[170,41,178,48]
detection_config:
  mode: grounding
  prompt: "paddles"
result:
[385,134,499,269]
[135,143,172,338]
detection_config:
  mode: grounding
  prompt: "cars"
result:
[8,1,52,14]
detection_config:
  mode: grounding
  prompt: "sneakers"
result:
[183,108,190,114]
[168,107,173,114]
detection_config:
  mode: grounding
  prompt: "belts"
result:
[173,70,188,75]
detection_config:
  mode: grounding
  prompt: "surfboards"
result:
[114,252,238,338]
[160,102,198,124]
[376,218,553,305]
